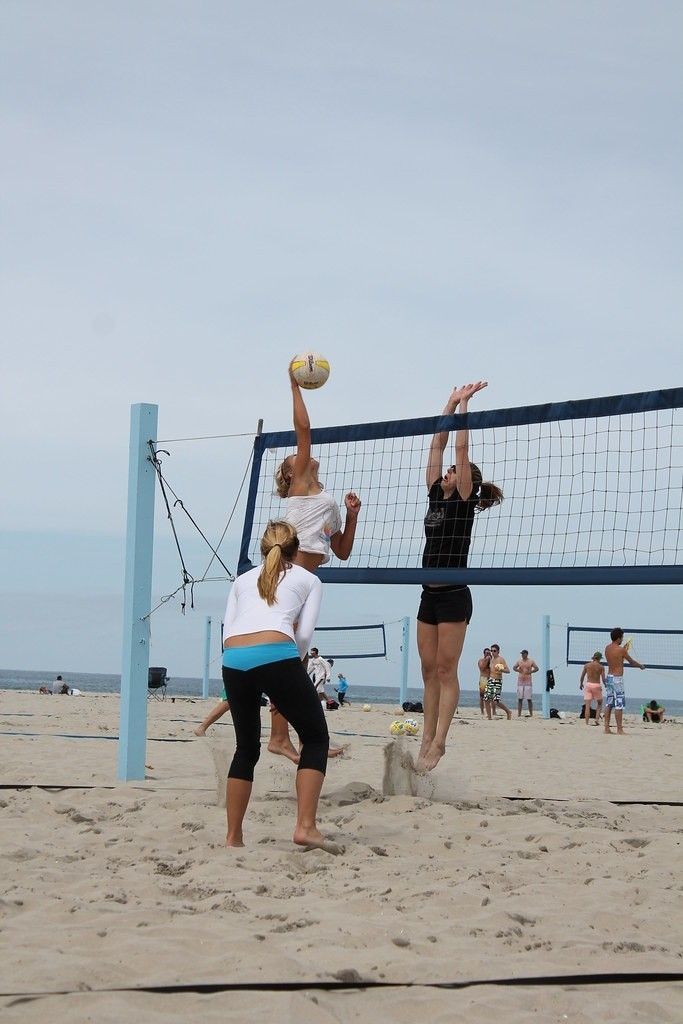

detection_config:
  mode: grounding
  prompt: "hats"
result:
[594,652,604,659]
[519,650,528,654]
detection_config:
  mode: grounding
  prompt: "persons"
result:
[52,676,69,695]
[579,652,605,724]
[478,648,498,715]
[225,519,344,855]
[415,381,504,771]
[641,700,665,723]
[483,644,511,720]
[337,673,351,707]
[513,650,539,717]
[307,647,334,702]
[268,355,361,764]
[604,628,645,735]
[194,685,230,736]
[39,684,52,694]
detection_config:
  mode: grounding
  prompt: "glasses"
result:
[451,465,456,473]
[491,650,497,652]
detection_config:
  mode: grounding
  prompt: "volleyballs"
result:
[389,720,406,736]
[494,663,505,674]
[363,704,372,712]
[291,350,331,390]
[403,718,420,736]
